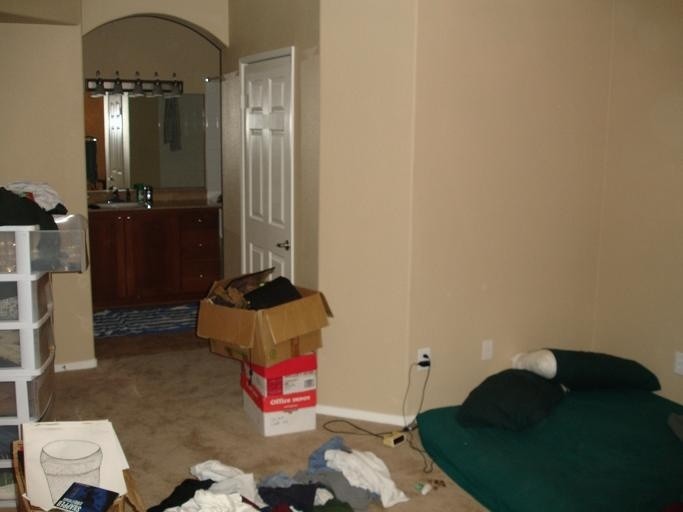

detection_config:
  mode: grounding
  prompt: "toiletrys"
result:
[122,183,154,210]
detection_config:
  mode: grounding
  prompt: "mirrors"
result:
[85,88,208,208]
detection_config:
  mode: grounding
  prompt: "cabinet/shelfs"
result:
[0,215,92,509]
[88,208,220,308]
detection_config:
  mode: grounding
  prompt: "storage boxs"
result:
[194,273,333,369]
[10,436,146,511]
[240,388,317,438]
[240,348,317,411]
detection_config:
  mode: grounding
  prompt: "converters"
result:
[417,361,431,367]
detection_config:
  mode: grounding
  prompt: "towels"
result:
[163,96,184,153]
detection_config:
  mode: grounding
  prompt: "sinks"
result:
[96,202,139,208]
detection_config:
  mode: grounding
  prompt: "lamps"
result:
[85,69,184,99]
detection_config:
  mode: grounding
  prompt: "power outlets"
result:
[416,347,432,371]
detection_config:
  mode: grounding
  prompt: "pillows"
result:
[510,346,662,392]
[455,367,571,429]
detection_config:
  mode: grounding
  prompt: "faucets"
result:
[108,190,124,202]
